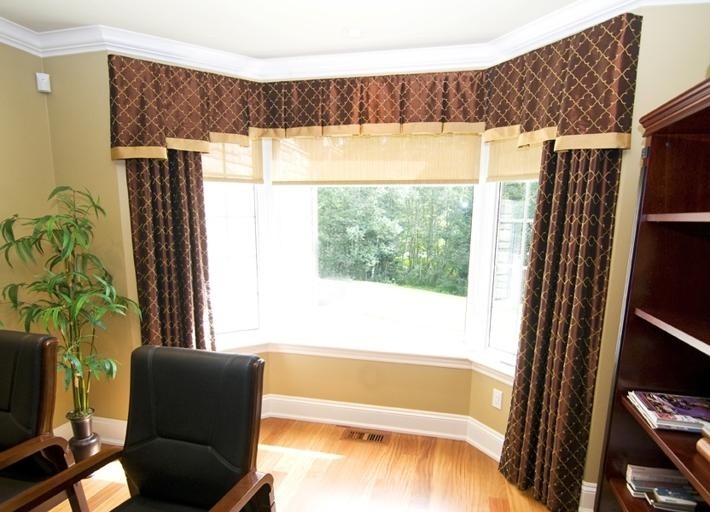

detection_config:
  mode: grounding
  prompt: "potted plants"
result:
[0,183,143,479]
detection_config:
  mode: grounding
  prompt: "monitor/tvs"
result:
[626,388,710,512]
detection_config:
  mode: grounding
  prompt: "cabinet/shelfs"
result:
[591,78,710,511]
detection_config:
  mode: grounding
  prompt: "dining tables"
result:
[0,183,143,479]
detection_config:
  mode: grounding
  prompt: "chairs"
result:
[4,345,275,512]
[2,331,86,510]
